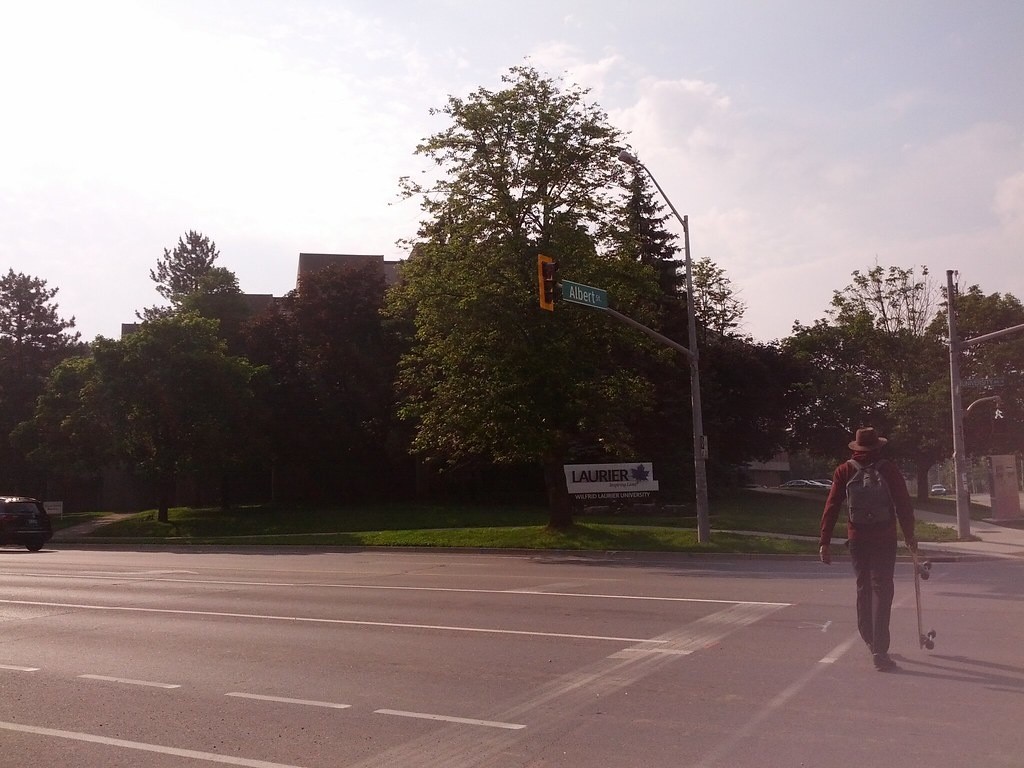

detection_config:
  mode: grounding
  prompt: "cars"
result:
[777,478,833,488]
[930,484,947,496]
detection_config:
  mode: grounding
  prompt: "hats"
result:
[847,427,888,451]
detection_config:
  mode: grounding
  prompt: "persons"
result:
[818,426,919,672]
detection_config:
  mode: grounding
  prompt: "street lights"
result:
[618,151,711,549]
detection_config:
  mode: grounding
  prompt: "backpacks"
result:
[845,458,895,527]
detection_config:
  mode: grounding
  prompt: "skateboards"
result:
[910,545,937,650]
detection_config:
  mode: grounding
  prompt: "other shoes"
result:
[873,649,900,674]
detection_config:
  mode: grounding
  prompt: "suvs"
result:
[0,495,54,553]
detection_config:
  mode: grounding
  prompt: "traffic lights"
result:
[538,253,560,310]
[991,406,1008,434]
